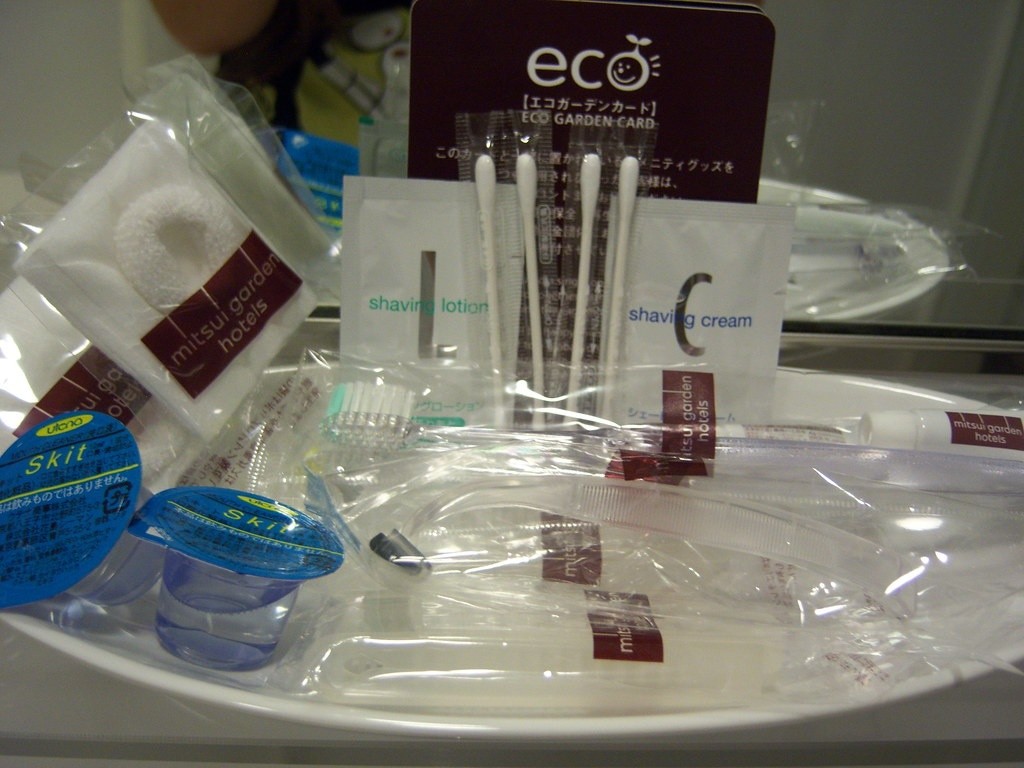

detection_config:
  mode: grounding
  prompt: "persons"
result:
[148,0,415,140]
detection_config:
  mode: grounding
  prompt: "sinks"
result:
[0,369,1024,758]
[316,178,953,325]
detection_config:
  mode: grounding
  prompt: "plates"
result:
[752,177,948,322]
[0,365,1022,752]
